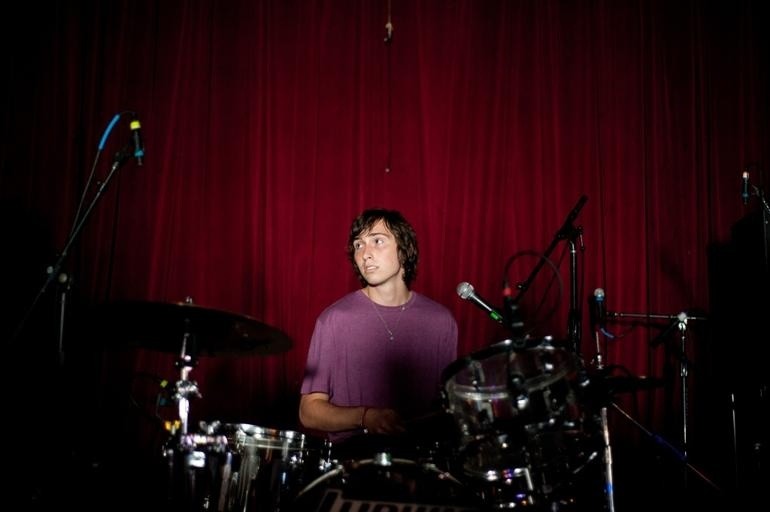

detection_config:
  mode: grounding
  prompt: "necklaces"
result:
[367,285,410,342]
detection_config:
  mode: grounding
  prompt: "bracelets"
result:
[360,403,370,430]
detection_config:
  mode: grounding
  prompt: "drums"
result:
[462,432,591,512]
[191,422,331,512]
[299,452,464,511]
[438,344,570,428]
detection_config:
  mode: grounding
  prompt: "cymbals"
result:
[103,297,293,358]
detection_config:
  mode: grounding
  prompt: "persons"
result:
[296,207,459,460]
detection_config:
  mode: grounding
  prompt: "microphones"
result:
[594,288,604,328]
[130,120,144,166]
[742,171,749,205]
[457,282,503,322]
